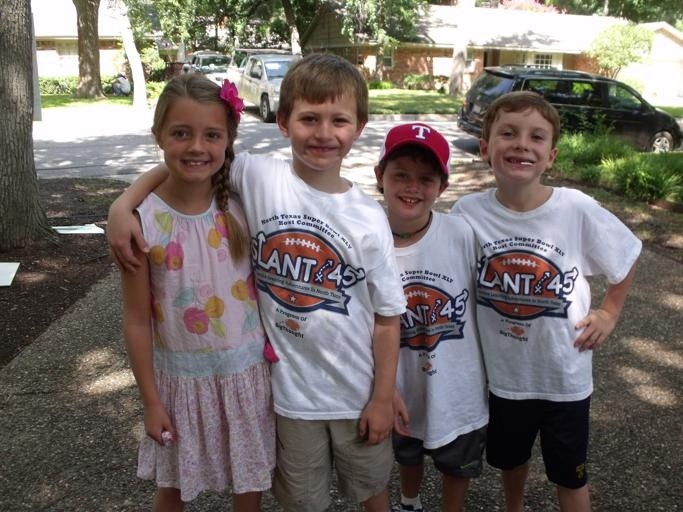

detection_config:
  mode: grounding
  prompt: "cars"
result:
[182,66,230,91]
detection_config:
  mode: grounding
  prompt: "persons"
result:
[374,122,491,512]
[449,91,643,512]
[106,52,407,511]
[122,73,277,512]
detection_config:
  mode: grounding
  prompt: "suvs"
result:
[458,61,682,161]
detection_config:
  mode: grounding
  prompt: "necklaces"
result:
[384,210,433,241]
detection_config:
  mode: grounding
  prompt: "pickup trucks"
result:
[223,50,319,119]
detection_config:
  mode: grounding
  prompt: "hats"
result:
[379,123,451,176]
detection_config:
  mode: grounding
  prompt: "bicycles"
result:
[103,80,122,98]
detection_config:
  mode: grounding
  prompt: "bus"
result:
[191,54,232,74]
